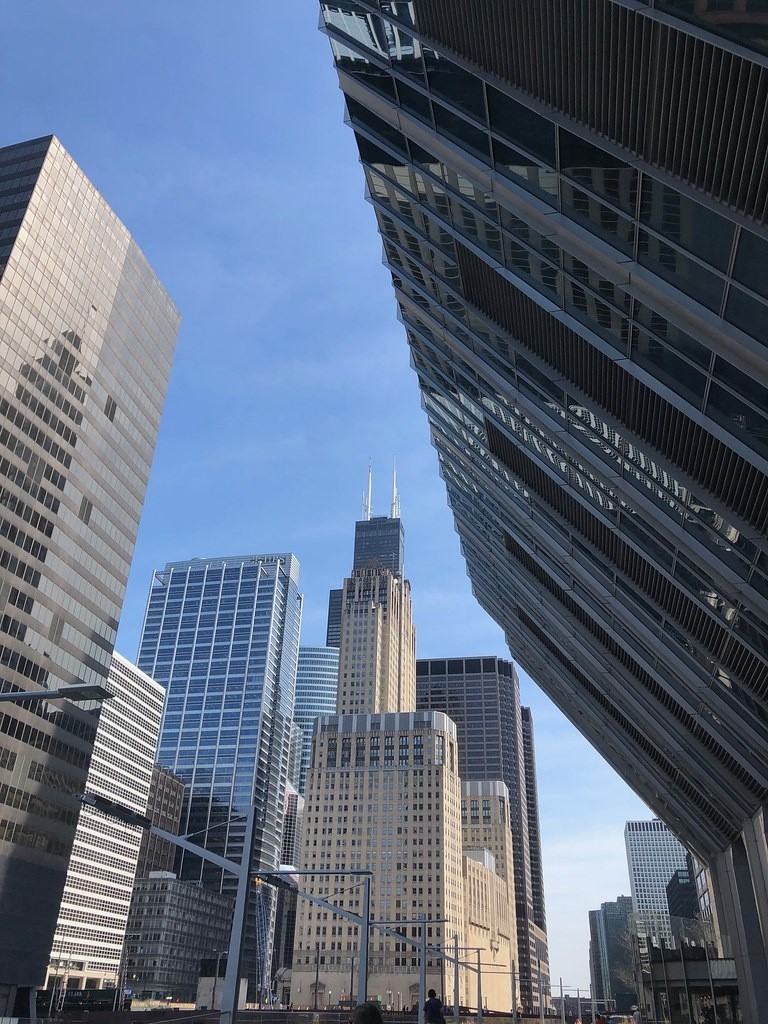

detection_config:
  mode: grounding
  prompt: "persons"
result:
[595,1014,604,1024]
[633,1009,641,1024]
[347,1004,384,1024]
[422,988,446,1024]
